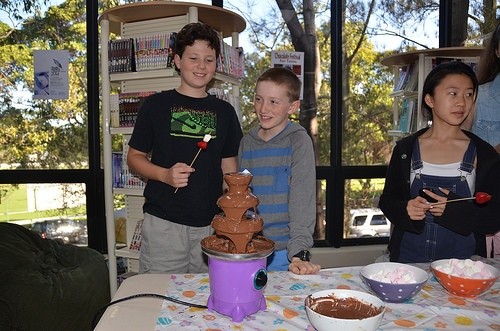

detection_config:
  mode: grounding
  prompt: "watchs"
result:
[293,249,311,262]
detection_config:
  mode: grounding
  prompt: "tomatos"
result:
[197,142,207,149]
[475,192,491,203]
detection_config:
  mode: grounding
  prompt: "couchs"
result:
[0,221,111,331]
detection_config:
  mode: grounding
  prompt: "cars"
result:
[348,208,392,238]
[28,219,88,246]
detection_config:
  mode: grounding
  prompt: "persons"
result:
[236,67,322,275]
[127,21,243,274]
[460,22,500,152]
[377,60,500,263]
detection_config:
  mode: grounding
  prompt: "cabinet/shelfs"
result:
[380,47,486,150]
[97,1,246,301]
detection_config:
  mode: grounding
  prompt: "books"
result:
[392,64,418,135]
[108,32,242,252]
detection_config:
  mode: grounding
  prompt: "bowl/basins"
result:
[359,262,430,303]
[305,289,386,331]
[430,259,500,298]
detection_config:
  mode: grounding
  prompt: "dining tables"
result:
[92,262,500,330]
